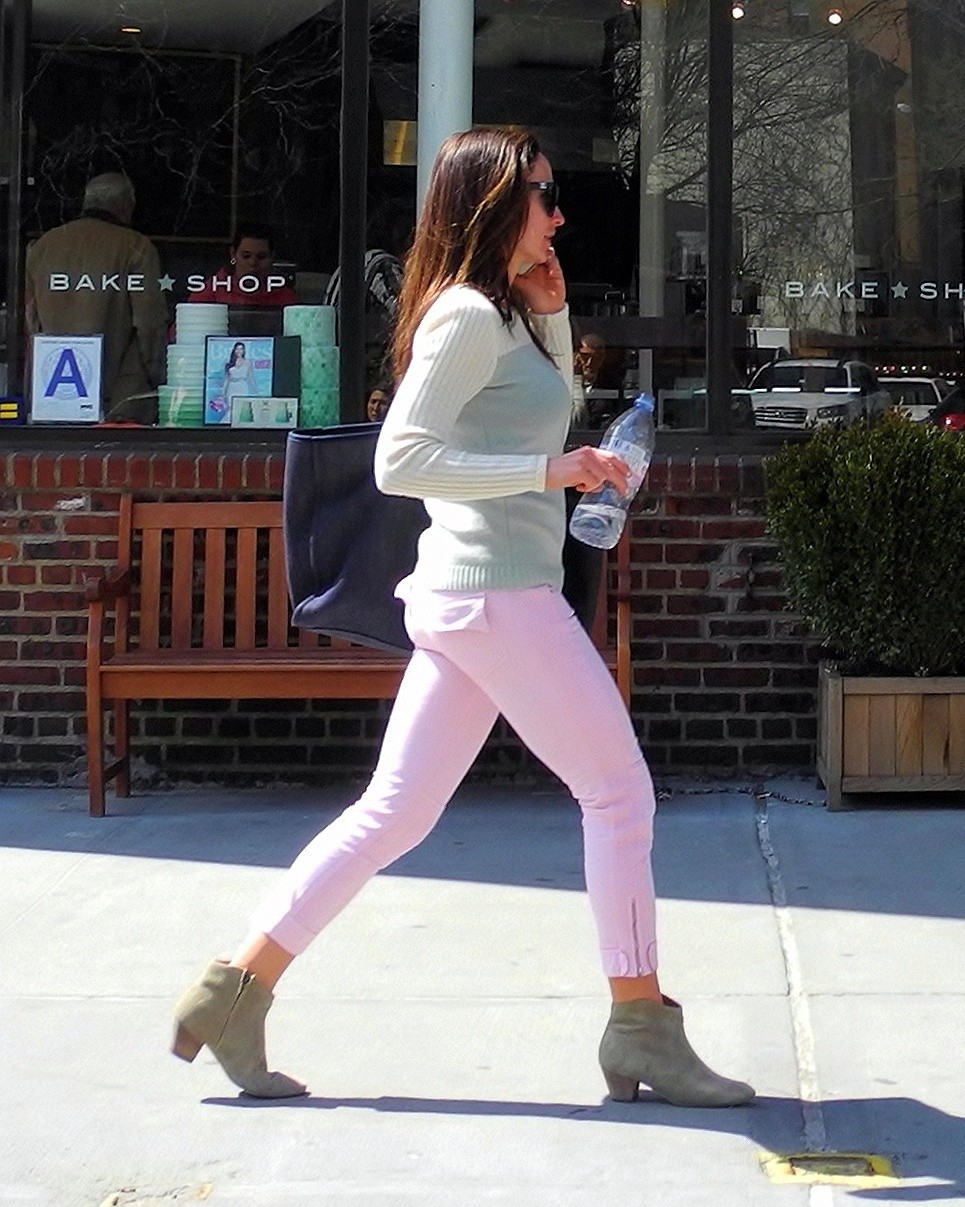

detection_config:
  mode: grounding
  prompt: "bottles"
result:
[568,390,657,552]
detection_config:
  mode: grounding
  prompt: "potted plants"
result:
[758,396,964,812]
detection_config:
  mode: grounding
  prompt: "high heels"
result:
[599,992,756,1108]
[170,957,307,1097]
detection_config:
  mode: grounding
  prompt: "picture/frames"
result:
[203,335,276,427]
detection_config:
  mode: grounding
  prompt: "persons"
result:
[171,219,297,343]
[24,174,168,422]
[569,325,626,430]
[220,343,259,422]
[169,126,758,1108]
[366,382,394,423]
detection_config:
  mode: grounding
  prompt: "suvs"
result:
[745,347,893,432]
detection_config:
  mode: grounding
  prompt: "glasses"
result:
[528,180,559,218]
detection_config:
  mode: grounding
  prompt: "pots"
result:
[591,291,638,317]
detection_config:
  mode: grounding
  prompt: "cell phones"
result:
[518,262,537,276]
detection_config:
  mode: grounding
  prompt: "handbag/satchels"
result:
[282,422,415,654]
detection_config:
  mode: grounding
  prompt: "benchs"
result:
[83,492,631,819]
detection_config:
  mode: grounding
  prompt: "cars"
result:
[876,376,949,420]
[918,384,965,432]
